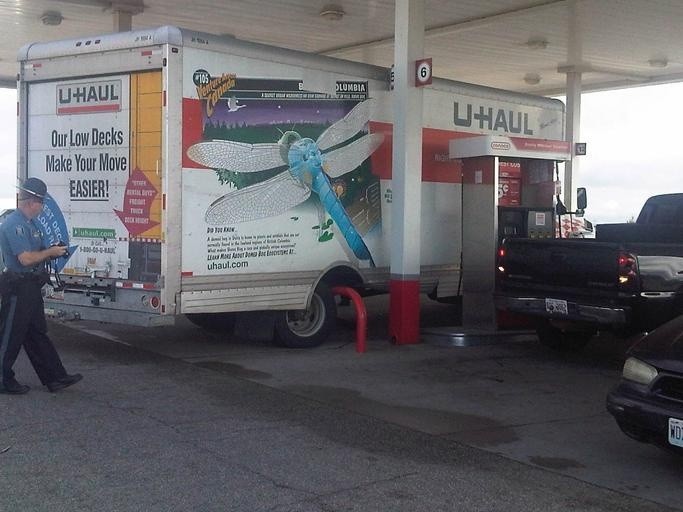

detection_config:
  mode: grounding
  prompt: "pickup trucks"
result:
[493,192,682,355]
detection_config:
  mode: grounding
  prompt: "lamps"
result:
[319,9,345,22]
[525,40,549,49]
[646,59,668,68]
[38,11,64,25]
[523,76,542,84]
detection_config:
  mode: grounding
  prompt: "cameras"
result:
[57,239,70,260]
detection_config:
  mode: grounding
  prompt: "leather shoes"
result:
[46,373,82,392]
[0,382,30,395]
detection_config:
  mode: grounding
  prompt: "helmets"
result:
[16,177,50,200]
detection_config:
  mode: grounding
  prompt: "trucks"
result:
[13,24,593,348]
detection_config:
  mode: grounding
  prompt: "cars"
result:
[605,316,682,462]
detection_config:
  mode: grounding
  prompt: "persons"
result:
[1,177,84,394]
